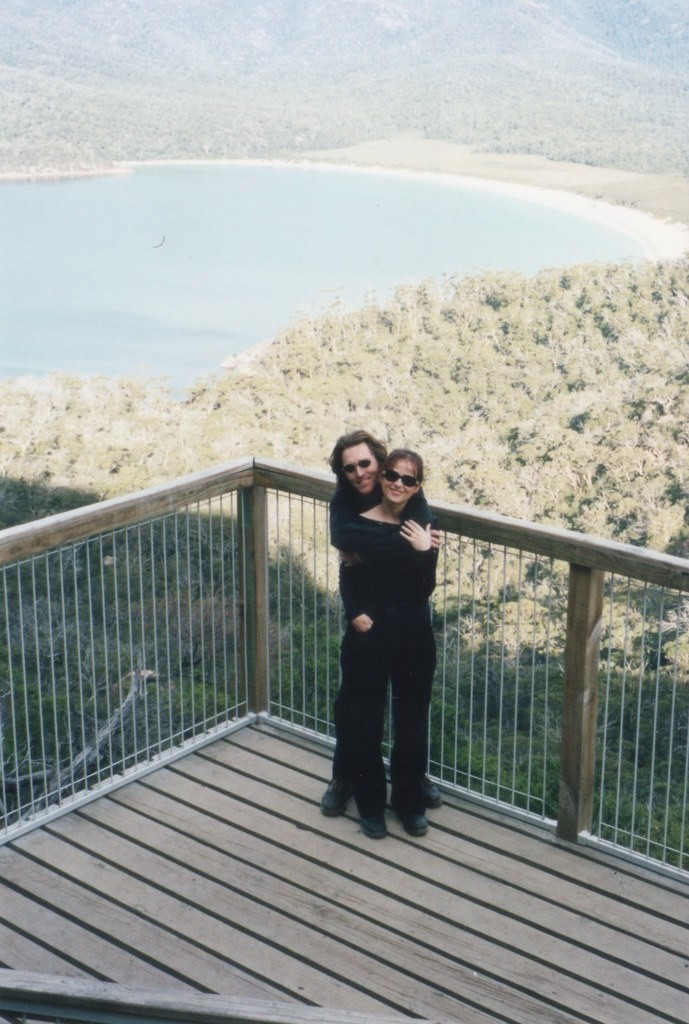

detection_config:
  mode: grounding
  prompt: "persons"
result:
[320,431,441,841]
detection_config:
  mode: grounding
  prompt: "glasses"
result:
[383,469,420,487]
[342,459,377,474]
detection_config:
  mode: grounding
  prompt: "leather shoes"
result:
[396,811,428,837]
[321,778,354,817]
[421,775,442,809]
[360,813,387,839]
